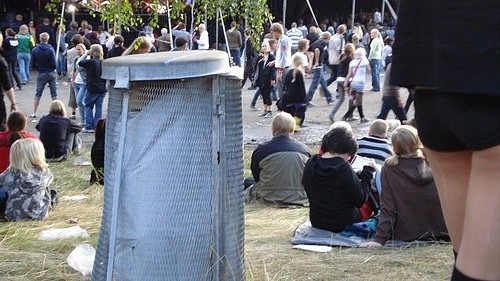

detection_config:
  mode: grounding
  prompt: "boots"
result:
[450,248,500,281]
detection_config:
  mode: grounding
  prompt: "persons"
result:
[170,20,209,51]
[318,8,414,123]
[35,100,82,162]
[76,44,108,132]
[329,118,429,209]
[226,18,334,126]
[0,112,59,222]
[360,125,453,248]
[138,23,171,52]
[0,32,18,125]
[121,36,152,57]
[90,117,106,186]
[387,0,500,281]
[28,33,57,118]
[243,110,313,208]
[8,14,128,85]
[68,34,87,127]
[302,126,381,235]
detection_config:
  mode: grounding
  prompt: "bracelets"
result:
[11,103,18,105]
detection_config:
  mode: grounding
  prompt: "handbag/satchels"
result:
[345,81,352,96]
[270,83,280,101]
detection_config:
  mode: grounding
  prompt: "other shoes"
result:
[67,115,76,119]
[259,111,272,118]
[346,116,359,121]
[328,113,335,125]
[81,126,95,133]
[360,117,370,123]
[50,189,59,206]
[28,112,36,118]
[306,101,317,108]
[328,100,336,106]
[77,122,87,127]
[249,106,260,111]
